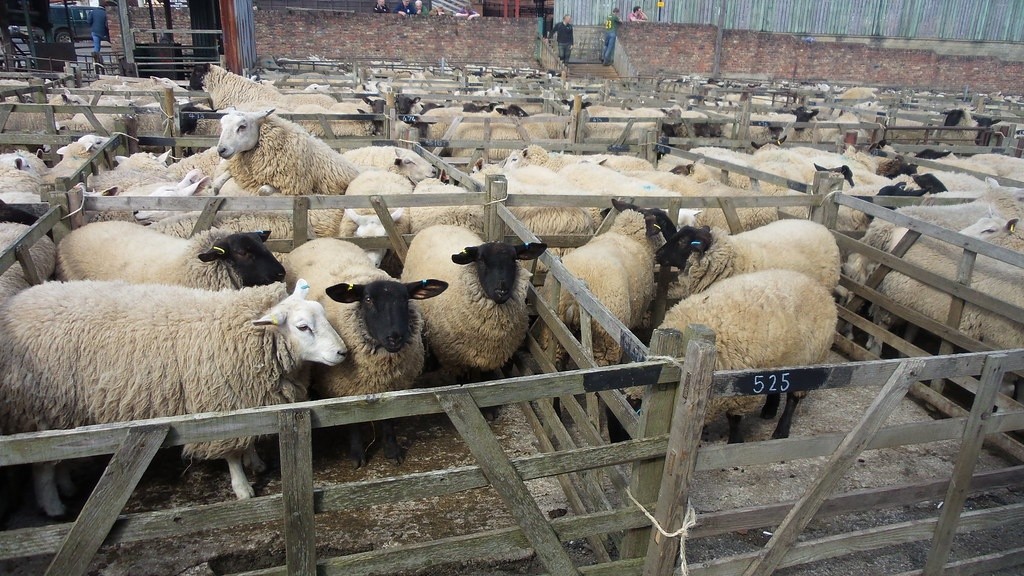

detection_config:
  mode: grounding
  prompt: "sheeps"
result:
[0,58,1024,524]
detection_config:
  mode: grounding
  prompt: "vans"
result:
[47,4,110,44]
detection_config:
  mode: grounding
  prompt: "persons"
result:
[628,6,648,22]
[429,5,454,17]
[600,8,623,66]
[86,4,111,54]
[543,14,574,67]
[373,0,391,13]
[393,0,427,16]
[456,5,480,21]
